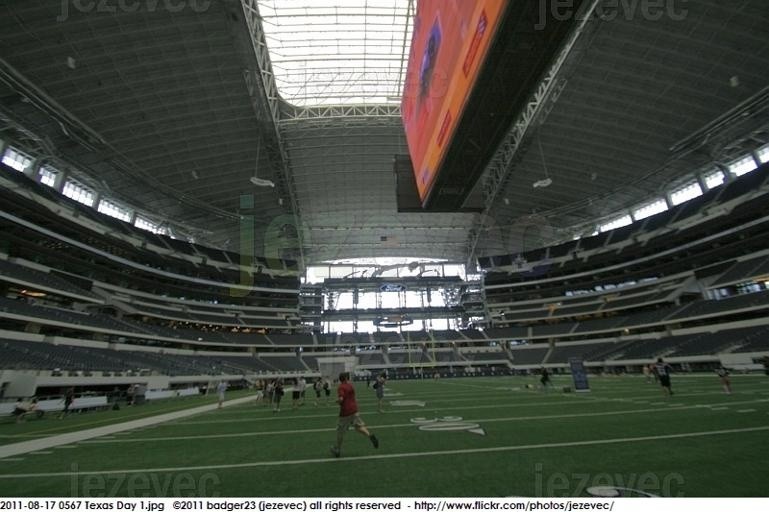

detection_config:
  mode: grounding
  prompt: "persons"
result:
[328,371,380,459]
[10,356,735,420]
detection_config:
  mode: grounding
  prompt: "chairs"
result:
[323,328,504,369]
[1,170,320,378]
[481,162,769,363]
